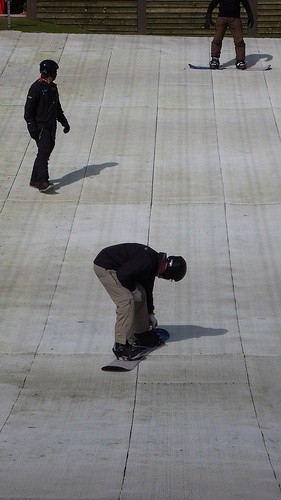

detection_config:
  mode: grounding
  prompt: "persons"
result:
[92,242,188,360]
[23,59,71,192]
[202,0,255,69]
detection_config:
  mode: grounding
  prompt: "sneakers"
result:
[134,333,161,346]
[112,344,142,360]
[235,60,246,70]
[209,59,219,68]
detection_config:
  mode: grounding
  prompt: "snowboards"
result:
[100,327,170,373]
[187,63,271,71]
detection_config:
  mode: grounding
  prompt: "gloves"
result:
[59,115,71,133]
[148,314,157,329]
[28,125,41,141]
[131,288,142,303]
[247,19,254,29]
[205,18,213,29]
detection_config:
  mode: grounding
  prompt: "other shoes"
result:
[31,181,56,192]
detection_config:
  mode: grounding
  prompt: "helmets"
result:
[40,59,58,76]
[158,256,187,282]
